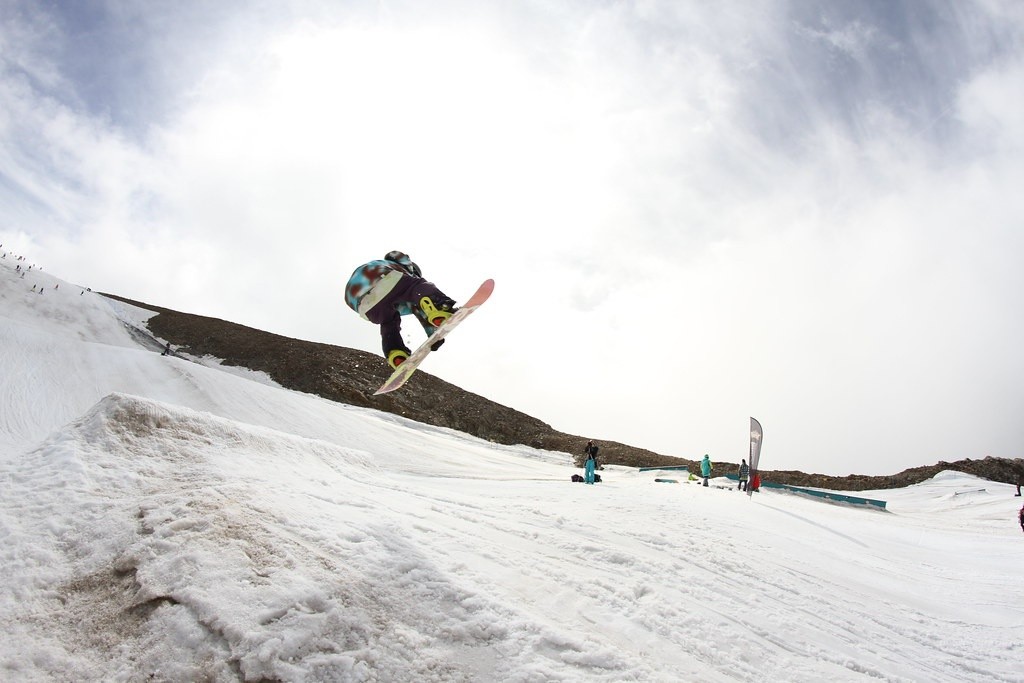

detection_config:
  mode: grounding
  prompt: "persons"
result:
[585,440,599,484]
[753,473,760,492]
[700,454,713,486]
[345,250,459,372]
[738,459,748,491]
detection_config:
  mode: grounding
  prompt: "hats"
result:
[704,454,709,459]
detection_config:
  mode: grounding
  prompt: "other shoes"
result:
[419,296,458,326]
[387,349,410,371]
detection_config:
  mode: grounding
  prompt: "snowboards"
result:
[373,279,495,396]
